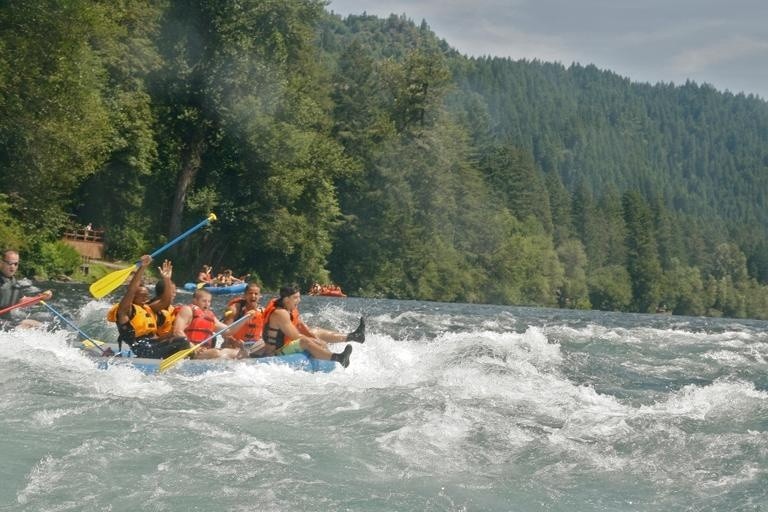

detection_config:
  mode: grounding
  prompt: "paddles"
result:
[196,277,217,290]
[40,299,114,358]
[89,212,218,301]
[159,307,263,372]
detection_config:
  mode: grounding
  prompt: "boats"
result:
[181,280,253,296]
[69,344,327,386]
[311,289,345,301]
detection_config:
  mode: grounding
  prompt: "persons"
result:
[212,272,224,288]
[234,272,251,286]
[147,278,183,338]
[221,281,268,358]
[0,249,54,333]
[219,269,242,287]
[262,284,366,369]
[308,281,347,297]
[198,265,215,287]
[83,222,93,241]
[173,288,246,360]
[114,254,195,360]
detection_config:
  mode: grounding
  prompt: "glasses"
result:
[3,259,18,267]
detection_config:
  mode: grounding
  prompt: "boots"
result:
[346,317,365,343]
[331,344,352,368]
